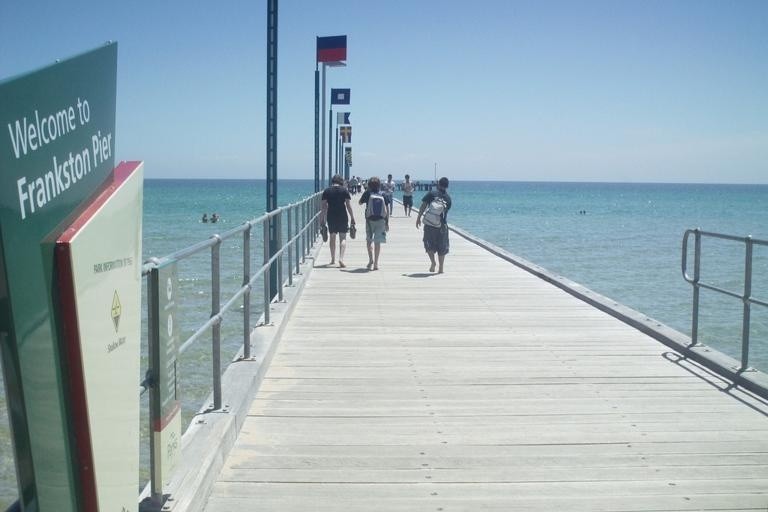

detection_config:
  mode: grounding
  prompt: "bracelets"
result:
[350,219,354,222]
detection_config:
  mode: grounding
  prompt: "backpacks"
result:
[366,191,387,218]
[422,191,447,228]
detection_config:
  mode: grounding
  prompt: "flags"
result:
[316,34,353,168]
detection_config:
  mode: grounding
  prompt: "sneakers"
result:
[321,226,327,241]
[350,225,356,238]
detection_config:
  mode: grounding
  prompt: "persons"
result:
[414,177,450,272]
[346,175,414,217]
[363,179,367,190]
[209,214,220,224]
[319,174,354,266]
[579,211,587,215]
[202,213,208,223]
[358,177,389,270]
